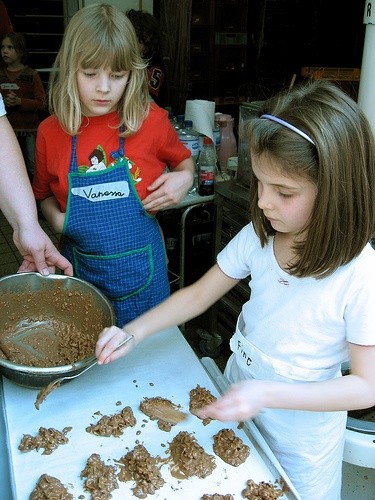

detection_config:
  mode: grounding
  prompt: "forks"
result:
[36,337,132,402]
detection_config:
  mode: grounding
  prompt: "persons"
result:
[0,10,168,187]
[94,76,374,500]
[33,3,195,329]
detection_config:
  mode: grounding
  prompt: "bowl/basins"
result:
[0,273,115,389]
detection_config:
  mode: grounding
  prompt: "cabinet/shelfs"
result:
[159,193,215,331]
[191,0,248,114]
[211,181,253,353]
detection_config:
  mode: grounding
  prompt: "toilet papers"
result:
[185,100,215,142]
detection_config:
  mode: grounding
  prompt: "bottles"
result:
[211,113,223,154]
[164,107,174,126]
[197,138,215,196]
[178,121,199,169]
[216,115,237,171]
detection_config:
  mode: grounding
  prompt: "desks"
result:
[0,324,301,500]
